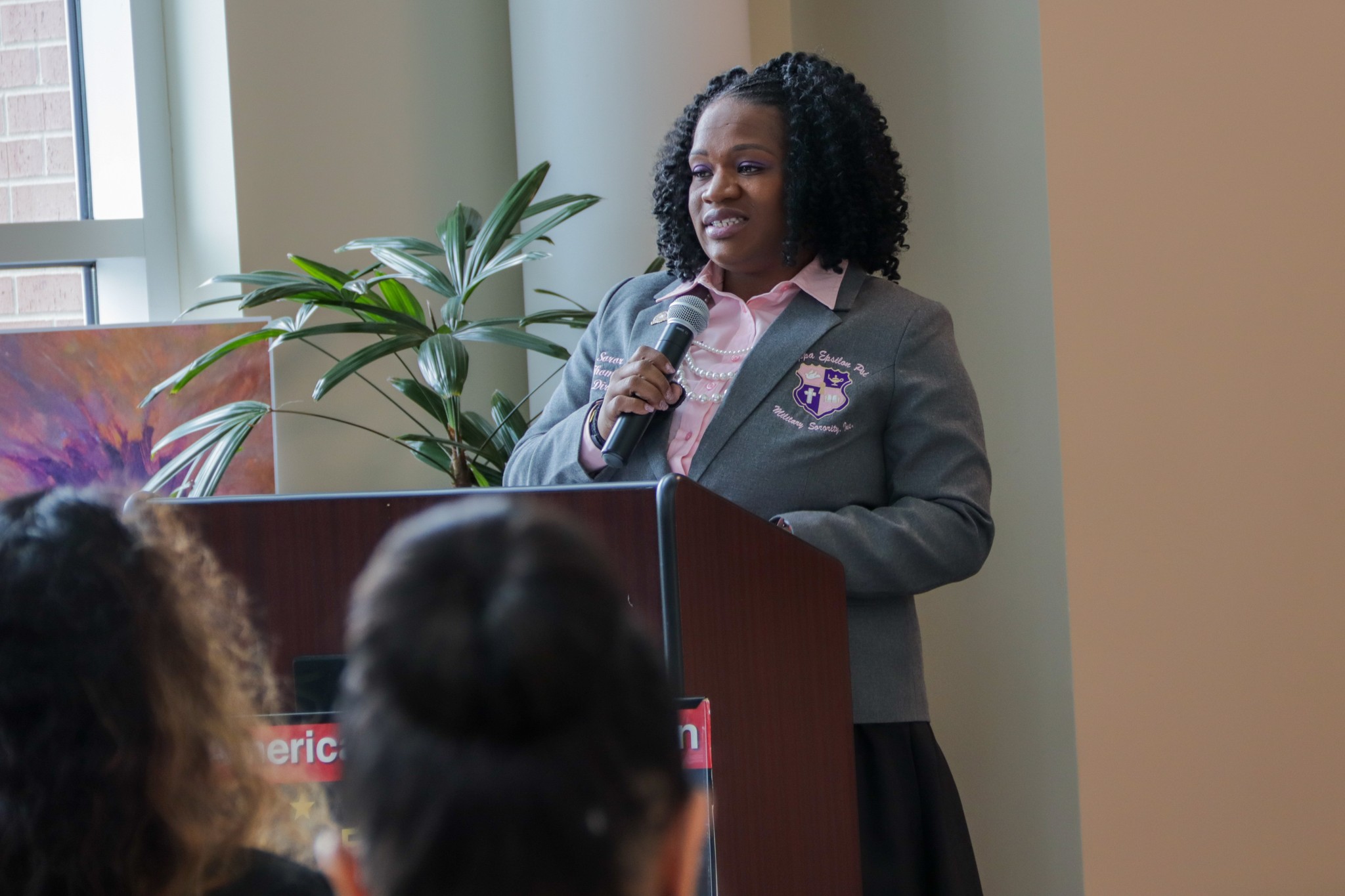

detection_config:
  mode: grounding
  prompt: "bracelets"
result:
[590,401,607,450]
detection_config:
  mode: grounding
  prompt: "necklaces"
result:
[674,339,754,405]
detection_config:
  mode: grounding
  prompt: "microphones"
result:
[602,295,710,469]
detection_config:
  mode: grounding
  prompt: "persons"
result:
[499,50,994,895]
[327,498,708,896]
[0,478,334,896]
